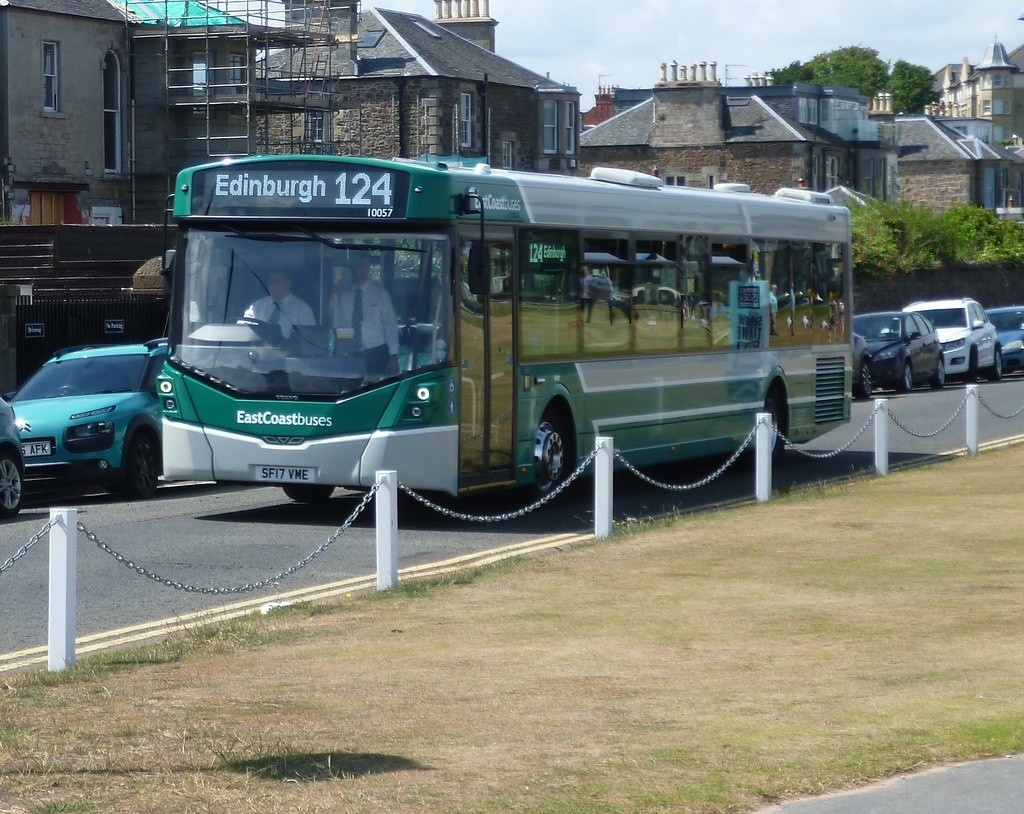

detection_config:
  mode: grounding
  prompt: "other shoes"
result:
[770,331,778,335]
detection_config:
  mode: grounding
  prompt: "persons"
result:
[769,285,846,345]
[234,268,316,338]
[576,267,695,327]
[331,246,402,373]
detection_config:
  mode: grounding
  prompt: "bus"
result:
[154,153,859,510]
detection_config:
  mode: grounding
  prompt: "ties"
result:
[272,301,280,324]
[351,288,363,349]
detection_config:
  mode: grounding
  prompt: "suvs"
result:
[901,298,1003,382]
[8,338,169,501]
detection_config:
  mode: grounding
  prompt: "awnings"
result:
[582,253,748,266]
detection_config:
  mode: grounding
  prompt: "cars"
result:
[853,312,945,393]
[853,332,873,400]
[0,398,26,518]
[984,306,1024,371]
